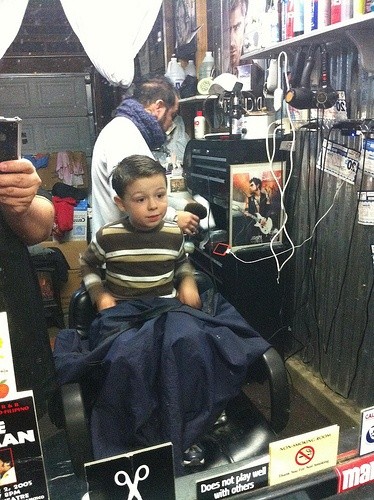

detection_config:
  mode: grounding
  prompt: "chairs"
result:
[68,268,289,477]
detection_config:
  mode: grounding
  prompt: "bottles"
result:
[202,52,214,79]
[194,111,205,139]
[166,58,185,89]
[185,60,196,77]
[229,92,241,139]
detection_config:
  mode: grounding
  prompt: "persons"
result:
[0,156,57,416]
[76,156,214,468]
[239,178,278,244]
[228,0,258,69]
[91,75,200,238]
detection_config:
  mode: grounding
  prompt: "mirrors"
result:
[0,0,374,500]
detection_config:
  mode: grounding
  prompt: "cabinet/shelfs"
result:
[240,0,374,61]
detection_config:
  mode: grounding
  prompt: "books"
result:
[264,0,374,49]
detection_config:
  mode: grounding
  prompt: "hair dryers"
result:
[285,56,318,109]
[313,52,338,109]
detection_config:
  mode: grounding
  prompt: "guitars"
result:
[237,206,274,235]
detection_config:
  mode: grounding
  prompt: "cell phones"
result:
[213,242,230,256]
[0,116,21,174]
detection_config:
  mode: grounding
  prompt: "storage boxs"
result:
[23,150,91,317]
